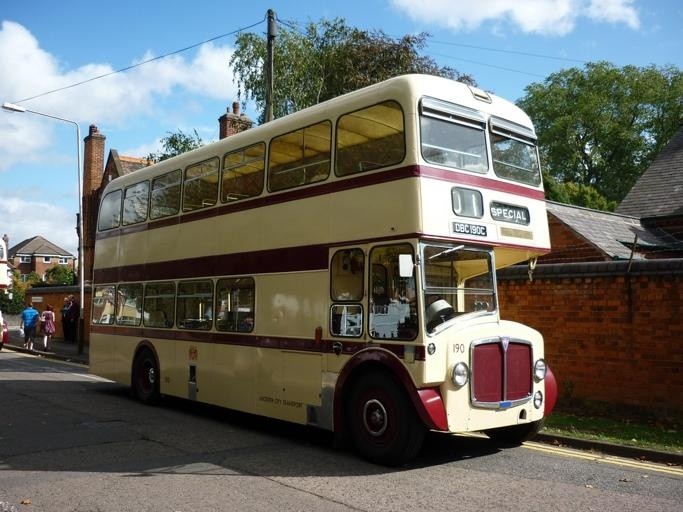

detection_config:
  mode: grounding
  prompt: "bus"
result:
[85,75,561,466]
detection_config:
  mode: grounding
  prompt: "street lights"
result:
[2,102,84,358]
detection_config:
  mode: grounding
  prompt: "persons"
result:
[20,303,42,350]
[59,294,80,343]
[40,305,57,352]
